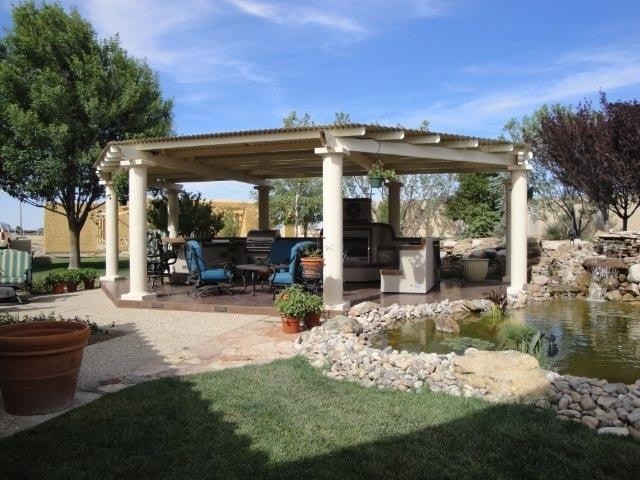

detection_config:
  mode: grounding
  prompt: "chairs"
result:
[146,231,234,297]
[0,249,34,292]
[268,241,317,300]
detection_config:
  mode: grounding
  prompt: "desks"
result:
[235,264,279,296]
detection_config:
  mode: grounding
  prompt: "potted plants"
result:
[301,247,324,279]
[367,160,395,187]
[274,287,325,334]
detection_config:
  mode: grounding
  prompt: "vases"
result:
[459,259,490,282]
[0,320,92,417]
[44,268,100,294]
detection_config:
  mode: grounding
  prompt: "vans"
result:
[0,222,18,249]
